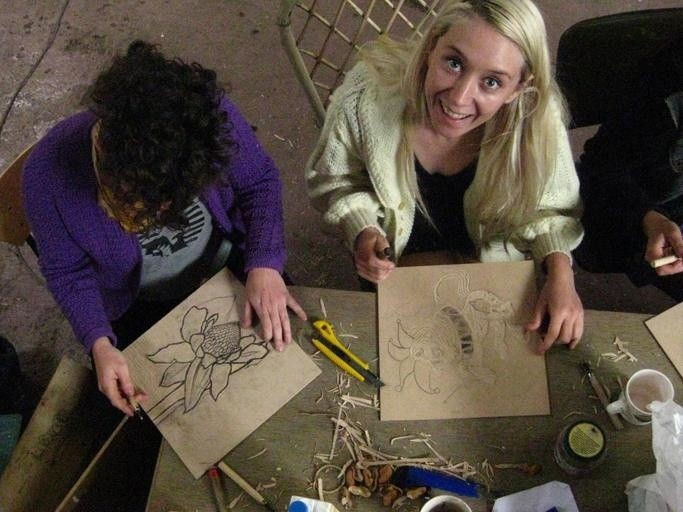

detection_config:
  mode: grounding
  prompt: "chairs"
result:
[282,1,460,118]
[0,133,45,258]
[557,9,681,128]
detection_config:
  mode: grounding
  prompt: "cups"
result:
[420,493,470,512]
[604,368,673,426]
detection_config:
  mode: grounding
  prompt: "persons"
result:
[23,40,307,416]
[304,0,586,354]
[574,55,683,303]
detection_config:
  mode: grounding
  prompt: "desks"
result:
[148,288,683,512]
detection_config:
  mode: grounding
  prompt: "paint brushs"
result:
[577,358,624,432]
[649,255,680,267]
[127,394,144,418]
[377,246,395,262]
[215,459,273,508]
